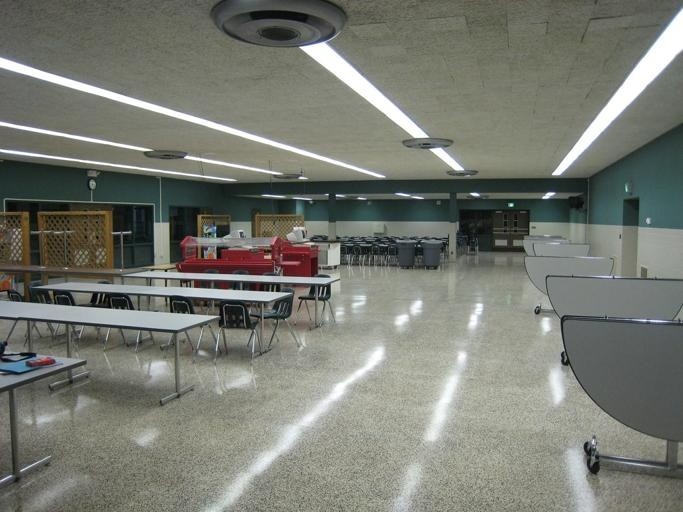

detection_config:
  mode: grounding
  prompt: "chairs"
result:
[341,246,397,267]
[5,281,301,362]
[200,267,336,327]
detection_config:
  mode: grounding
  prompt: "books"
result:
[0,353,64,375]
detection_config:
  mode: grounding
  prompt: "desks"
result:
[1,351,87,485]
[1,300,221,406]
[120,270,340,330]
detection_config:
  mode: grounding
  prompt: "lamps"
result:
[209,0,348,48]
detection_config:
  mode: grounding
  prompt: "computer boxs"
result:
[293,226,308,237]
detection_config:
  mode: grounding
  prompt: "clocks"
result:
[88,178,97,190]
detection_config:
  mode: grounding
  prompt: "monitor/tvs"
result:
[286,229,305,241]
[223,230,245,238]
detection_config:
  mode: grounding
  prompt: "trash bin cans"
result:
[420,240,443,270]
[397,240,418,269]
